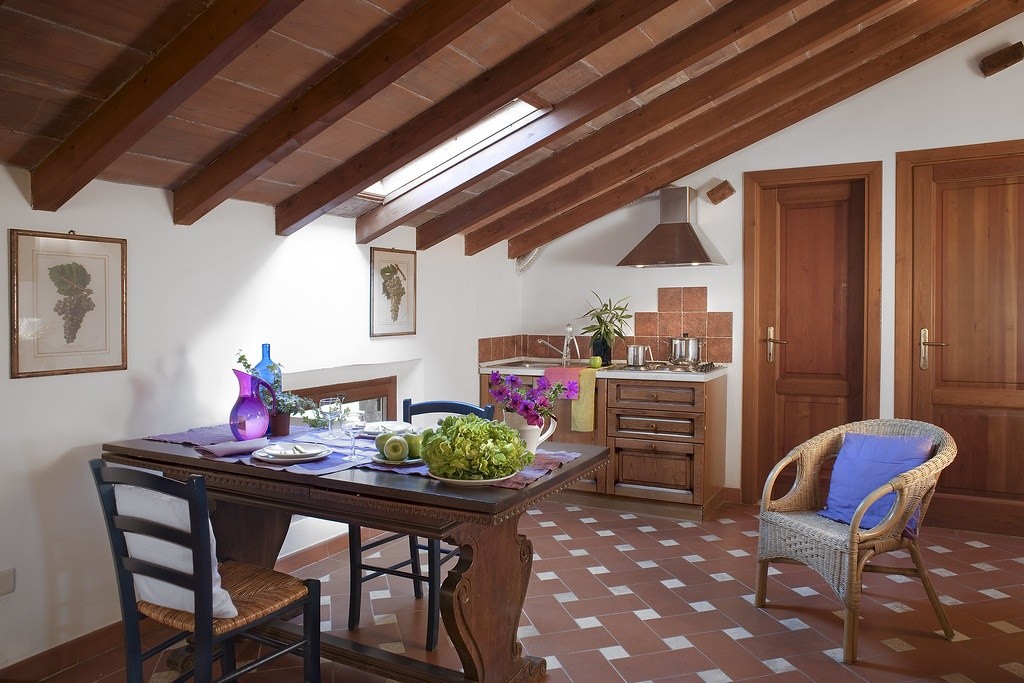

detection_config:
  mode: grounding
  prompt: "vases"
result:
[503,410,557,456]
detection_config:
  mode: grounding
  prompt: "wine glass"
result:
[319,398,341,440]
[341,413,366,461]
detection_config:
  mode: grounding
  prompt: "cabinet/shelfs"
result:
[480,373,727,521]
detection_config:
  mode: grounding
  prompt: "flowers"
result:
[488,370,579,427]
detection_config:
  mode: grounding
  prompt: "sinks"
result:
[487,359,592,370]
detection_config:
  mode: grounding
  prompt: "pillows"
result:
[113,483,238,618]
[817,430,932,541]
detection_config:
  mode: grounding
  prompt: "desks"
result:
[102,417,610,683]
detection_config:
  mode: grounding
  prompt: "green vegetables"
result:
[420,413,535,480]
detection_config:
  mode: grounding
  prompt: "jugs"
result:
[232,368,277,440]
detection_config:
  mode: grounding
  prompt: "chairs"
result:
[87,459,320,683]
[754,419,958,663]
[348,399,494,653]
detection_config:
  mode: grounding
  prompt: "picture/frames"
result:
[11,229,128,379]
[370,247,416,337]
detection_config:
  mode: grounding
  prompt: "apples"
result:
[590,356,602,368]
[374,429,424,461]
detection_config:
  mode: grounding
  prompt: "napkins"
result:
[193,437,270,456]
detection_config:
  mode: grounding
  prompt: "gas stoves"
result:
[605,358,724,374]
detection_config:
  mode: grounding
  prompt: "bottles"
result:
[252,344,282,396]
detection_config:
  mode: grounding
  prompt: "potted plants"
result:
[575,289,632,366]
[235,348,350,436]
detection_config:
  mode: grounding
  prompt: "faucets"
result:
[537,324,573,365]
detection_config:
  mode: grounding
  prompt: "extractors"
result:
[616,184,730,268]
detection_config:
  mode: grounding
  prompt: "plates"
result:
[372,451,424,464]
[427,471,518,486]
[363,420,410,434]
[252,444,330,464]
[264,443,328,458]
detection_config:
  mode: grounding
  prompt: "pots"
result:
[663,332,708,365]
[625,344,654,366]
